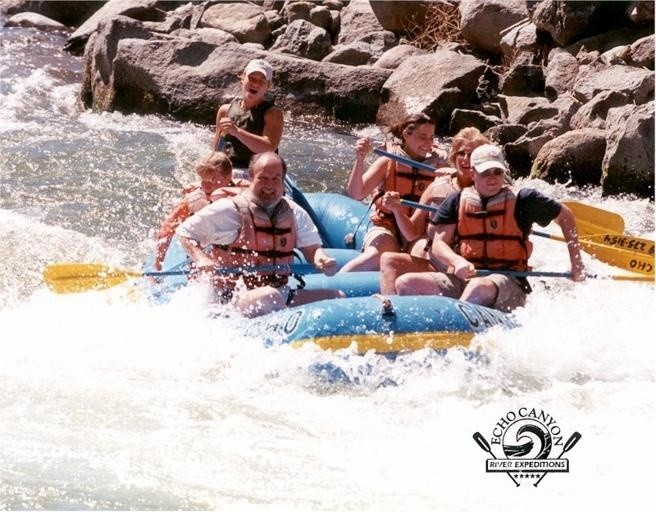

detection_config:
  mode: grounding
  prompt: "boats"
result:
[143,194,525,381]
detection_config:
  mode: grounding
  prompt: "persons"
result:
[148,150,250,297]
[394,144,584,313]
[210,60,282,169]
[174,149,346,319]
[379,126,491,296]
[337,113,456,272]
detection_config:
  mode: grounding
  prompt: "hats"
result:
[471,144,507,173]
[245,60,274,81]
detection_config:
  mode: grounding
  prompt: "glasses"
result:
[455,150,471,158]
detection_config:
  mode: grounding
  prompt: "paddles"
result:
[398,198,656,277]
[444,264,655,282]
[43,263,316,295]
[368,145,625,236]
